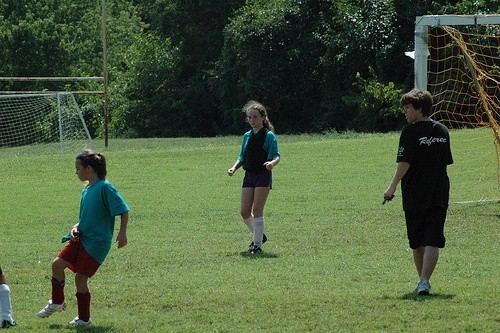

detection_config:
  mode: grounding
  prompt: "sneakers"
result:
[249,233,267,248]
[2,318,16,328]
[412,280,430,296]
[70,316,92,325]
[37,298,66,317]
[241,246,262,254]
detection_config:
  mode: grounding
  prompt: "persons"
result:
[38,150,129,327]
[228,101,281,255]
[0,269,18,327]
[382,89,453,294]
